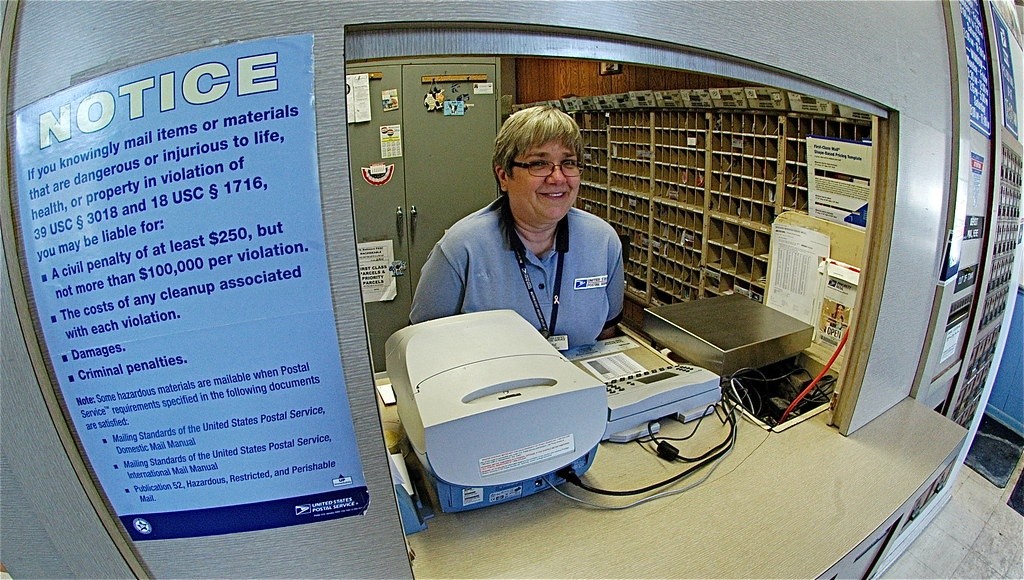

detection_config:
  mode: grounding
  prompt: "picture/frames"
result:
[599,62,622,76]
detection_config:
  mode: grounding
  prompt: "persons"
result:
[408,106,625,352]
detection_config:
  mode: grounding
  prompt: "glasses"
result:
[508,160,584,177]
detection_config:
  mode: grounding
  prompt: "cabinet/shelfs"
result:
[346,57,503,373]
[514,104,873,328]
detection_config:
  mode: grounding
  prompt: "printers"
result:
[385,310,609,514]
